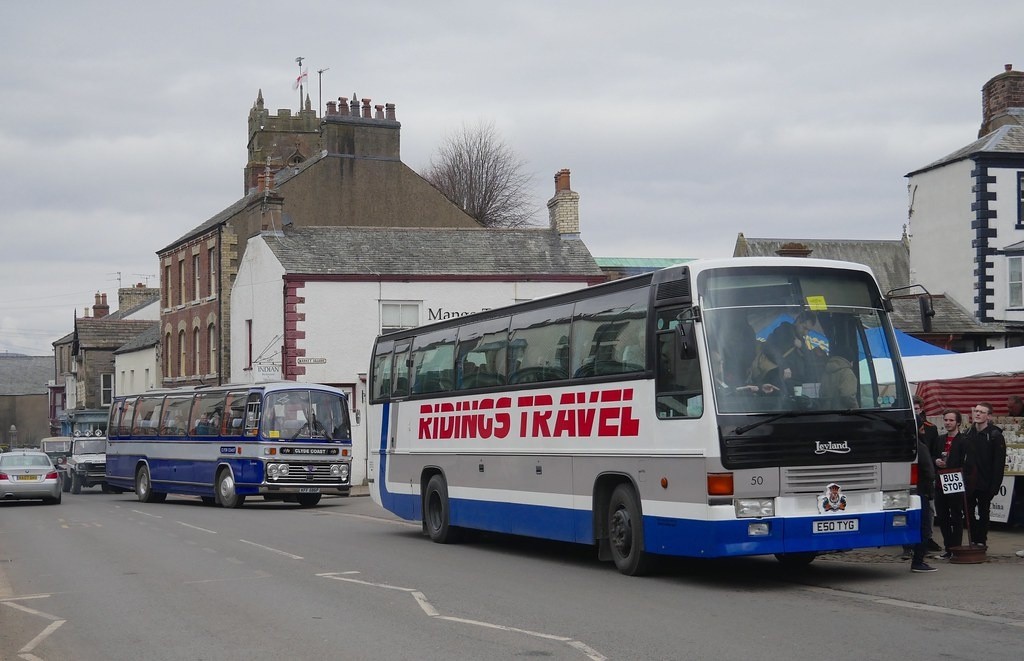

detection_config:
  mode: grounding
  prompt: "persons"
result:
[947,403,1007,564]
[707,296,859,409]
[910,413,938,573]
[136,409,221,437]
[934,410,971,559]
[902,394,942,554]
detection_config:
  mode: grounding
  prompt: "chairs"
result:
[111,405,314,442]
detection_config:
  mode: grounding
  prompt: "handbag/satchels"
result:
[750,350,764,380]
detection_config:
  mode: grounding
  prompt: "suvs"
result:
[40,437,70,466]
[60,428,122,494]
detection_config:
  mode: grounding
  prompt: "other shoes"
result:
[901,538,953,573]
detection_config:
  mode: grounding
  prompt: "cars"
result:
[0,451,62,505]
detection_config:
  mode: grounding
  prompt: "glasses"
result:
[973,409,989,414]
[915,408,920,410]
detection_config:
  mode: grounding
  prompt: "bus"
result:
[104,382,362,508]
[366,255,935,577]
